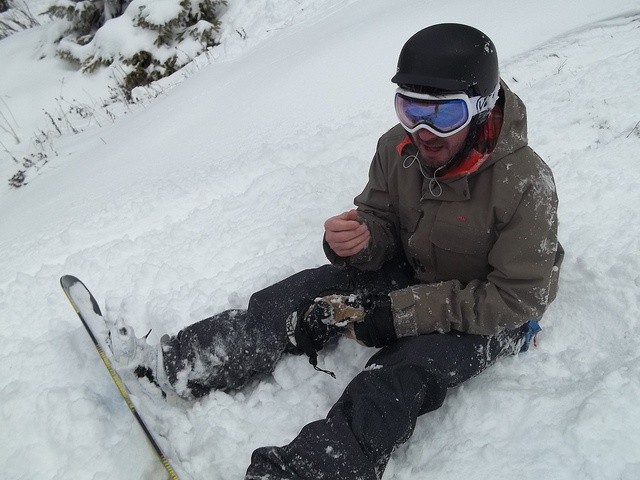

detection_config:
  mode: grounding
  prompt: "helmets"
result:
[391,22,499,98]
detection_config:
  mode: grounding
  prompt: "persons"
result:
[106,23,565,480]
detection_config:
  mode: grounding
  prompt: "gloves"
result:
[352,293,397,348]
[285,295,367,379]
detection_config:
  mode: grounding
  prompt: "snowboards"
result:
[59,275,181,480]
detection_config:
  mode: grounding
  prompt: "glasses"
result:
[394,89,498,138]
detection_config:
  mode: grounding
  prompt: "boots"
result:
[107,321,196,414]
